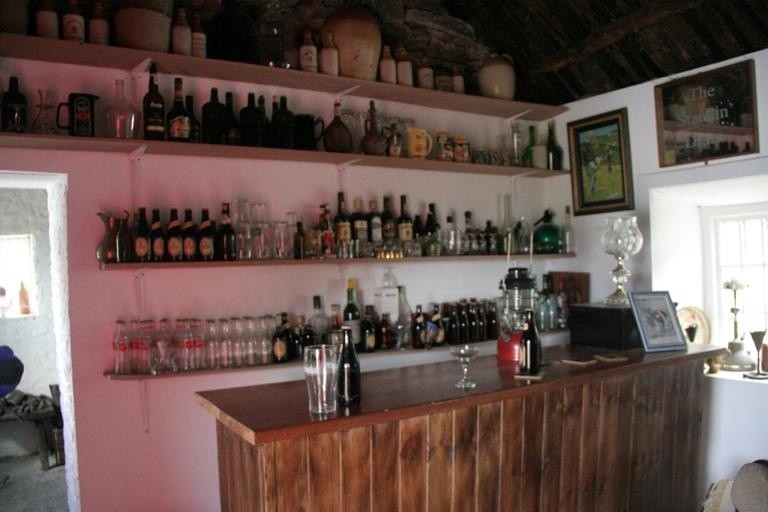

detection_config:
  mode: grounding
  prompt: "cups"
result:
[303,344,338,415]
[55,93,100,136]
[335,326,363,404]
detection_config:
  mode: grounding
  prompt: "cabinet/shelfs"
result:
[1,30,575,381]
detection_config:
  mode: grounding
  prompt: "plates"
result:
[677,304,713,348]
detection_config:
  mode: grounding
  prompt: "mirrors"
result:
[0,170,82,512]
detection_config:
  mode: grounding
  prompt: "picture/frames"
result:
[629,290,687,353]
[654,59,759,169]
[566,107,634,216]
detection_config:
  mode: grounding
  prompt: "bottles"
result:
[30,88,55,135]
[95,192,576,260]
[108,273,571,372]
[266,2,517,99]
[170,1,207,60]
[19,280,31,314]
[4,77,29,133]
[33,1,111,47]
[117,73,561,171]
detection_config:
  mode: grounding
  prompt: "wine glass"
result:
[748,327,768,379]
[449,340,481,389]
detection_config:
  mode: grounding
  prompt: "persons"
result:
[580,132,613,198]
